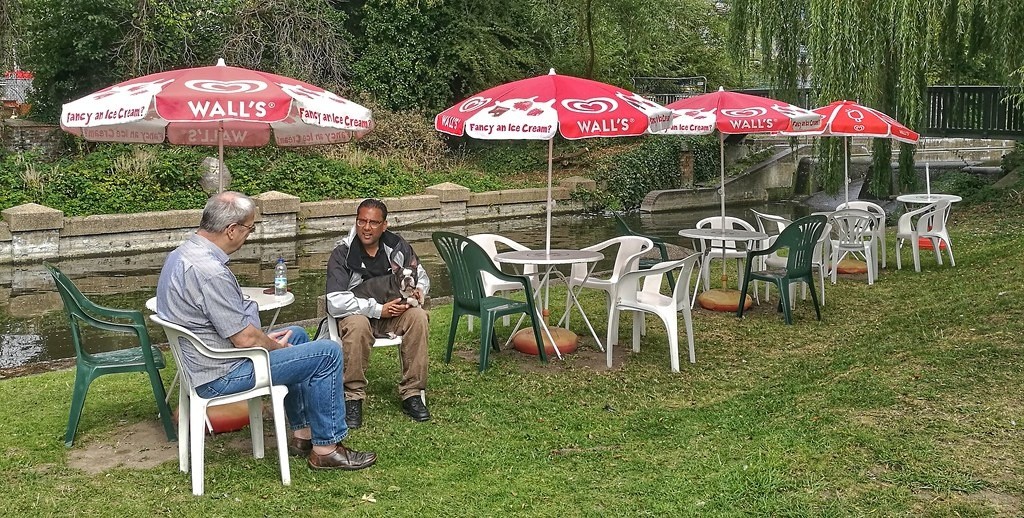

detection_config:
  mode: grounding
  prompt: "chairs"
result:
[326,298,426,406]
[429,230,548,374]
[44,260,293,498]
[562,197,956,376]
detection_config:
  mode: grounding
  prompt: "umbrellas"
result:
[434,67,673,356]
[644,85,824,313]
[779,99,920,274]
[60,59,372,194]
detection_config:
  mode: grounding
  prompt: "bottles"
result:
[274,259,287,296]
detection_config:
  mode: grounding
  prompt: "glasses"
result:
[355,216,384,229]
[226,222,256,233]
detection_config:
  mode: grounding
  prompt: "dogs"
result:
[349,254,423,340]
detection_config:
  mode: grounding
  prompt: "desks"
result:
[896,193,963,249]
[809,210,885,275]
[676,227,769,310]
[496,248,607,363]
[144,284,295,442]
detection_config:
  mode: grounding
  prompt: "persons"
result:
[156,191,378,472]
[326,199,430,429]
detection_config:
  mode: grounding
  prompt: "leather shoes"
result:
[346,400,363,429]
[305,442,378,471]
[289,436,313,457]
[400,396,430,422]
